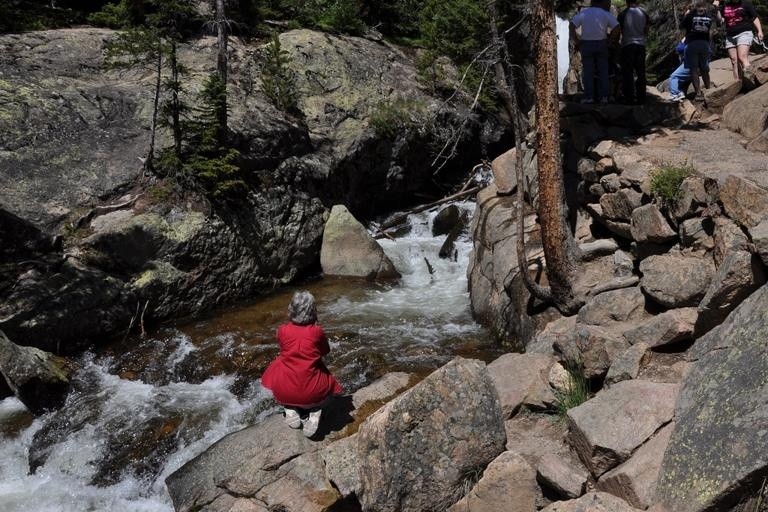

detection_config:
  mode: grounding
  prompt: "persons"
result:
[668,39,713,101]
[569,0,650,105]
[683,0,724,108]
[260,289,342,438]
[713,0,764,80]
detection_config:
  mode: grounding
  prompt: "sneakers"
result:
[598,96,608,105]
[303,407,323,438]
[579,96,594,105]
[283,404,301,429]
